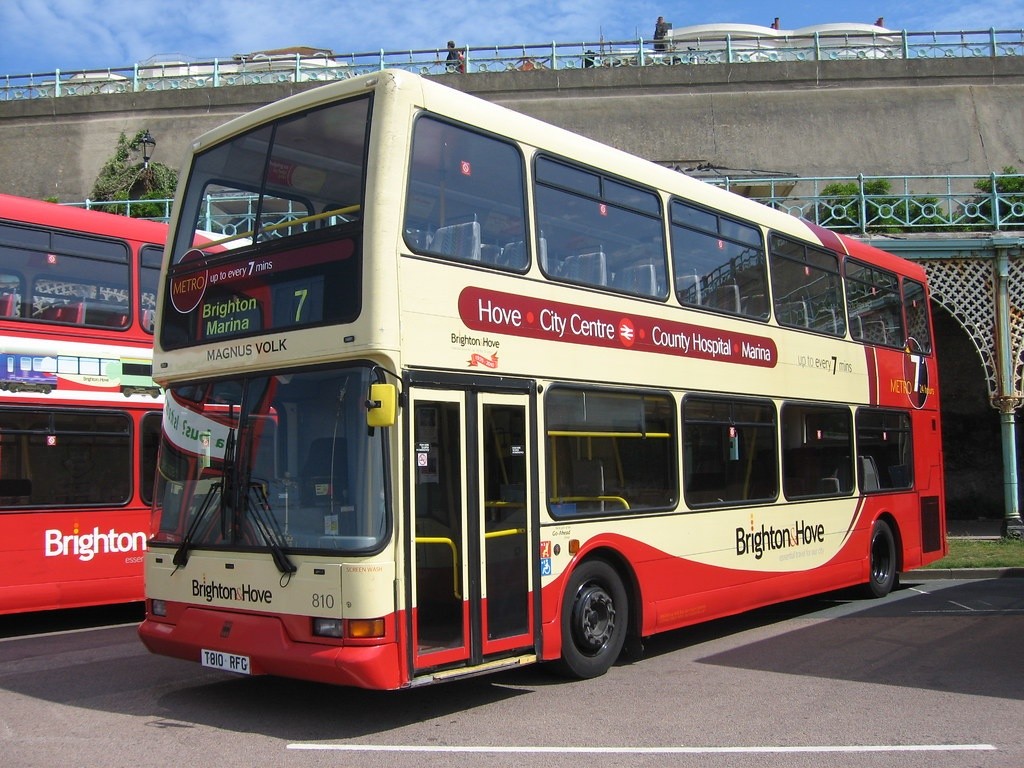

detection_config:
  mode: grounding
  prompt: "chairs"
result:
[299,437,351,508]
[0,294,152,331]
[320,204,902,347]
[489,440,899,510]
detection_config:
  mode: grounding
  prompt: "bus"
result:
[0,192,277,614]
[138,66,948,693]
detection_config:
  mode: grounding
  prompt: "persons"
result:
[446,41,459,70]
[457,50,465,73]
[654,17,670,51]
